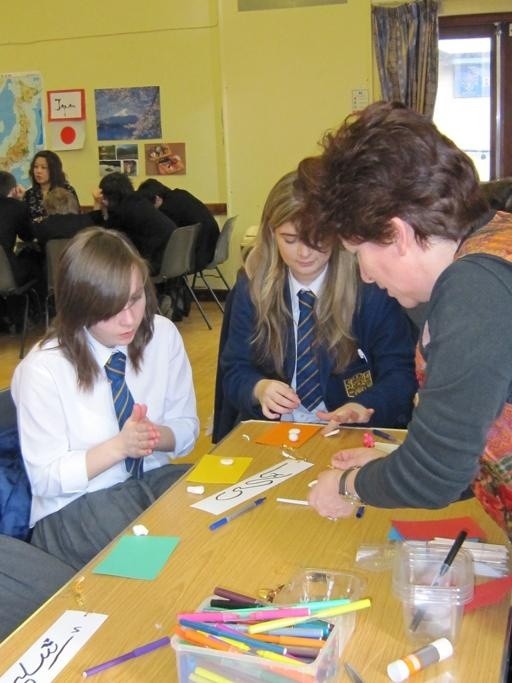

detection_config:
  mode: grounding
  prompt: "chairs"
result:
[192,216,238,314]
[146,223,214,330]
[45,237,73,330]
[0,245,44,359]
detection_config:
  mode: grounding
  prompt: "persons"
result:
[0,147,82,330]
[10,226,202,569]
[219,164,419,430]
[293,96,511,525]
[80,171,222,321]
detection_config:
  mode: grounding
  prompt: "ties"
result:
[295,289,323,413]
[104,351,145,480]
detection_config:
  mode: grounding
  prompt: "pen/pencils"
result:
[174,587,372,682]
[209,496,266,530]
[356,506,364,517]
[308,479,318,488]
[277,498,308,508]
[373,429,403,443]
[82,636,170,678]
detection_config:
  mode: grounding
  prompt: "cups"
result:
[282,565,362,642]
[393,541,475,641]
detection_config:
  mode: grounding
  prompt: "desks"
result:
[0,419,512,683]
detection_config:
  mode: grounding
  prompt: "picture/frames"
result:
[46,87,87,122]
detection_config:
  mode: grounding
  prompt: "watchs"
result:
[338,464,365,507]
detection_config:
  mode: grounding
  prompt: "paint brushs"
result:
[407,530,467,634]
